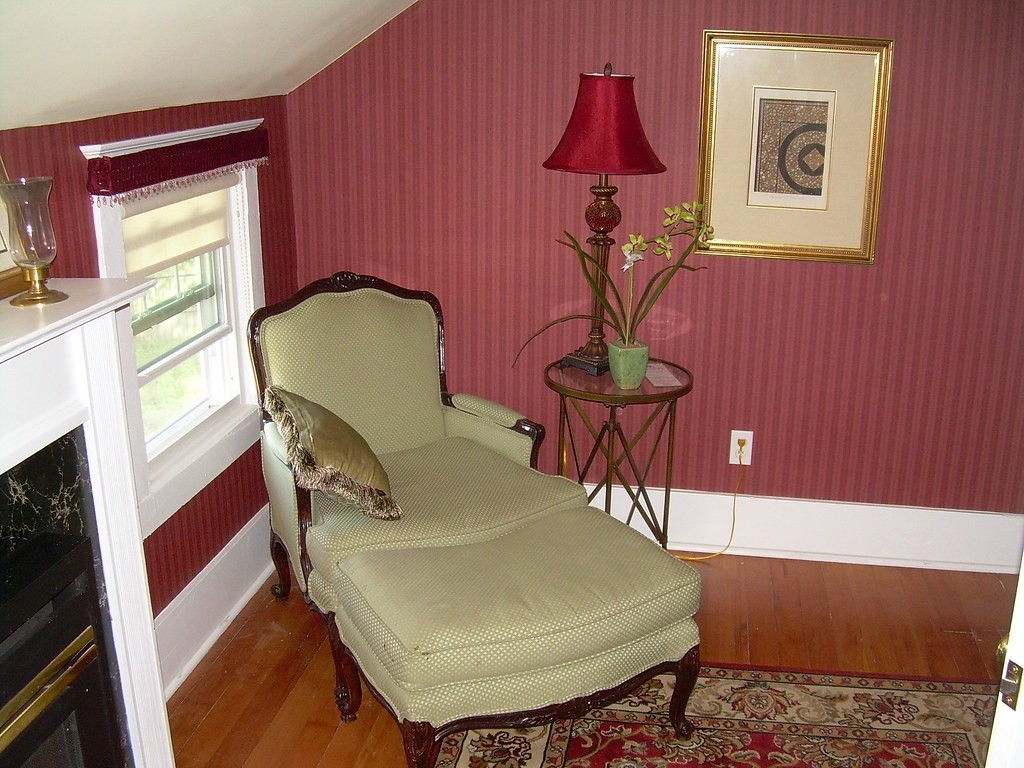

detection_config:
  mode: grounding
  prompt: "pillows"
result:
[262,384,404,521]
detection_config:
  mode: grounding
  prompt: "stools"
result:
[328,505,704,768]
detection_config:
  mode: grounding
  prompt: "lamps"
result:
[543,61,667,377]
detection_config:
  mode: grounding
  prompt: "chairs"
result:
[246,271,545,623]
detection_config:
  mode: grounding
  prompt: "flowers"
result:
[511,202,714,369]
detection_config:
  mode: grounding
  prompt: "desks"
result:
[544,357,694,550]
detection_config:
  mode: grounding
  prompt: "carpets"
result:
[429,661,1000,768]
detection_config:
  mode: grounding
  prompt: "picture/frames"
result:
[694,29,895,265]
[1,157,49,299]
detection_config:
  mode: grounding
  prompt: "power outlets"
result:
[729,430,754,465]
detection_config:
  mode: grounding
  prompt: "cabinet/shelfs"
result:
[0,277,175,768]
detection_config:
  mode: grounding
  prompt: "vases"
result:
[609,341,648,389]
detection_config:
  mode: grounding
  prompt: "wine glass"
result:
[0,175,70,307]
